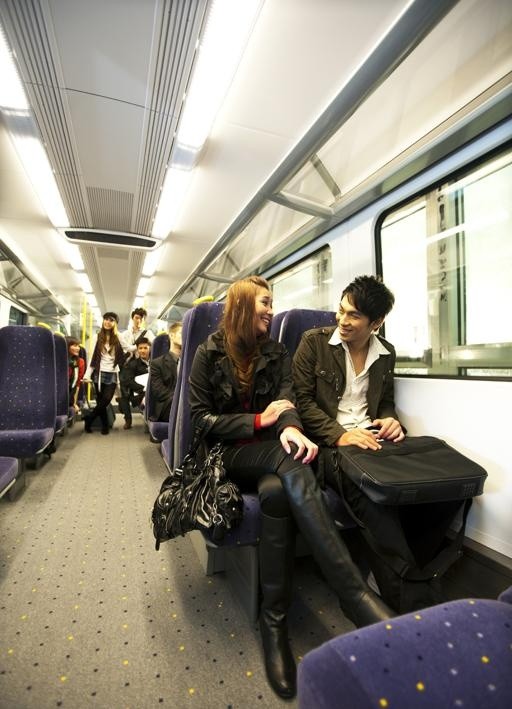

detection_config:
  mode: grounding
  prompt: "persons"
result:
[64,307,182,435]
[188,275,398,699]
[291,273,437,596]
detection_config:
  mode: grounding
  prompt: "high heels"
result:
[81,415,93,433]
[101,423,109,434]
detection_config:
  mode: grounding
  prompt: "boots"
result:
[258,507,297,699]
[279,463,400,629]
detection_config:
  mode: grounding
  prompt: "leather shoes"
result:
[427,578,448,605]
[116,396,124,412]
[123,418,132,429]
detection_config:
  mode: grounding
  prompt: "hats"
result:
[103,312,119,324]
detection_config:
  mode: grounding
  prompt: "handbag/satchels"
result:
[151,413,244,550]
[330,425,488,582]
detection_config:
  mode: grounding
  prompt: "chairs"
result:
[297,583,512,708]
[0,325,88,502]
